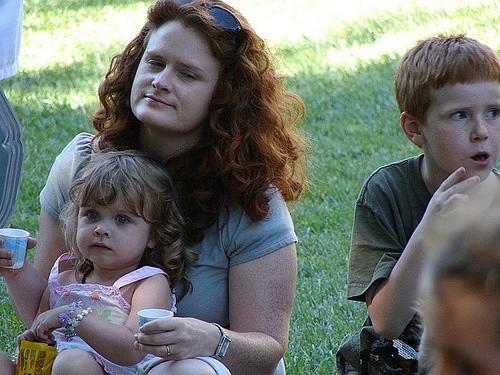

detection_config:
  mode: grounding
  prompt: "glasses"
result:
[176,0,244,32]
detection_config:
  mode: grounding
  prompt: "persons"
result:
[415,195,500,375]
[0,0,307,375]
[336,34,500,375]
[0,150,199,375]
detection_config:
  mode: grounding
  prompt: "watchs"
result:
[208,322,232,361]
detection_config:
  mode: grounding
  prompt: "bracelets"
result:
[58,300,83,342]
[68,307,92,334]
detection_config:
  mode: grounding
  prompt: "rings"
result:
[165,346,171,356]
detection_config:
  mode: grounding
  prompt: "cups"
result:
[0,228,32,268]
[137,309,174,334]
